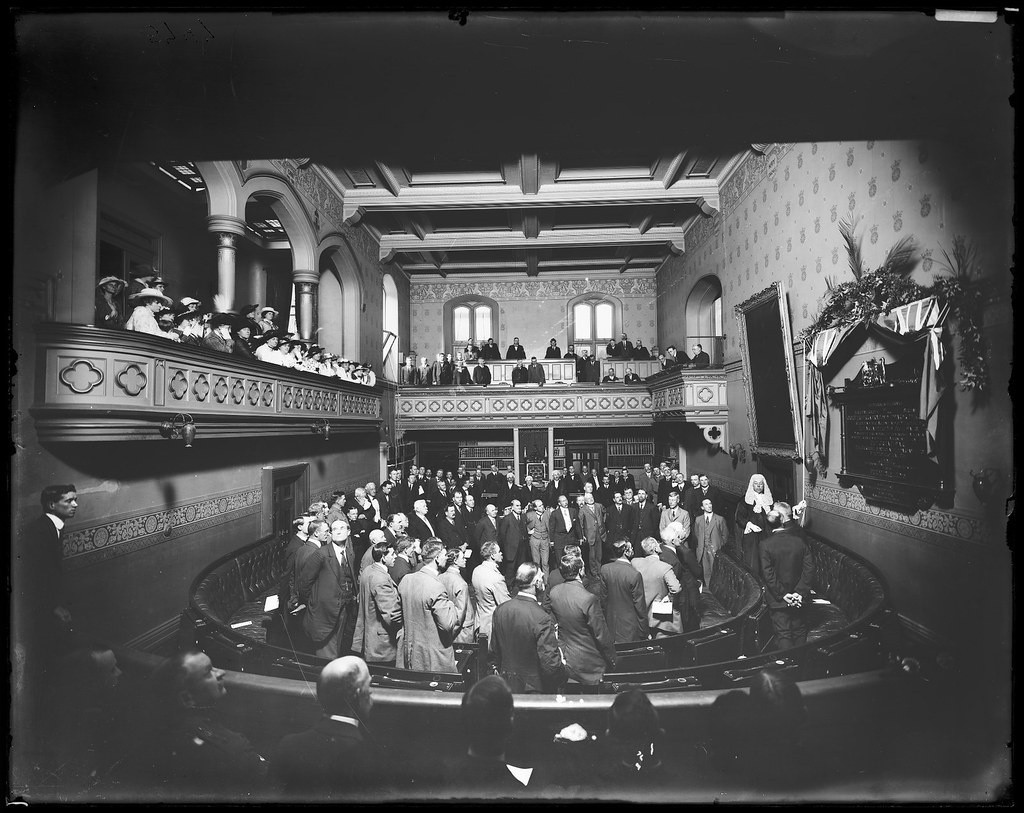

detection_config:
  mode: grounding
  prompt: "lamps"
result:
[970,468,999,506]
[159,412,196,447]
[729,443,746,464]
[804,451,827,479]
[311,418,330,440]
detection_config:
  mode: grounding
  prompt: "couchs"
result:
[178,492,892,695]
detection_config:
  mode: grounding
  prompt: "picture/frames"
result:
[734,281,805,461]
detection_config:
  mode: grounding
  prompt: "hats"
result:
[518,358,522,360]
[129,288,173,308]
[321,352,334,363]
[532,357,536,359]
[209,313,235,325]
[133,264,158,276]
[306,346,325,358]
[260,307,279,316]
[150,277,168,287]
[348,360,359,367]
[261,330,279,345]
[98,275,128,287]
[479,357,484,359]
[514,337,518,339]
[362,362,372,369]
[180,297,199,306]
[338,359,350,367]
[277,330,295,337]
[351,364,370,379]
[273,338,294,350]
[235,317,251,331]
[155,301,190,315]
[332,354,340,361]
[239,304,259,315]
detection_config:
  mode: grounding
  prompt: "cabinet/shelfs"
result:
[386,442,416,480]
[459,446,514,470]
[553,436,565,470]
[606,434,655,469]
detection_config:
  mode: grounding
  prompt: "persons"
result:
[471,540,512,647]
[19,273,987,790]
[694,498,729,586]
[436,504,463,548]
[392,540,459,673]
[473,504,501,549]
[438,548,475,643]
[406,499,437,549]
[549,495,584,568]
[498,499,528,594]
[526,499,551,587]
[631,536,684,640]
[351,543,403,662]
[598,539,652,644]
[488,561,570,693]
[658,528,691,634]
[667,521,703,578]
[296,519,357,659]
[548,554,620,688]
[759,510,815,651]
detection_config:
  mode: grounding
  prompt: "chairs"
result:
[525,457,547,483]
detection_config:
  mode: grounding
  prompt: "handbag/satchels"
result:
[651,595,672,614]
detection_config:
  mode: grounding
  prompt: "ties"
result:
[625,499,632,505]
[706,516,709,525]
[618,504,622,528]
[470,509,472,513]
[679,486,682,492]
[397,480,400,484]
[340,550,347,570]
[456,504,462,512]
[420,478,422,483]
[639,503,643,530]
[517,515,519,520]
[702,488,708,495]
[409,484,411,490]
[672,511,675,517]
[470,354,471,358]
[535,513,543,522]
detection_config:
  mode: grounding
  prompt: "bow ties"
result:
[373,497,377,500]
[401,532,407,534]
